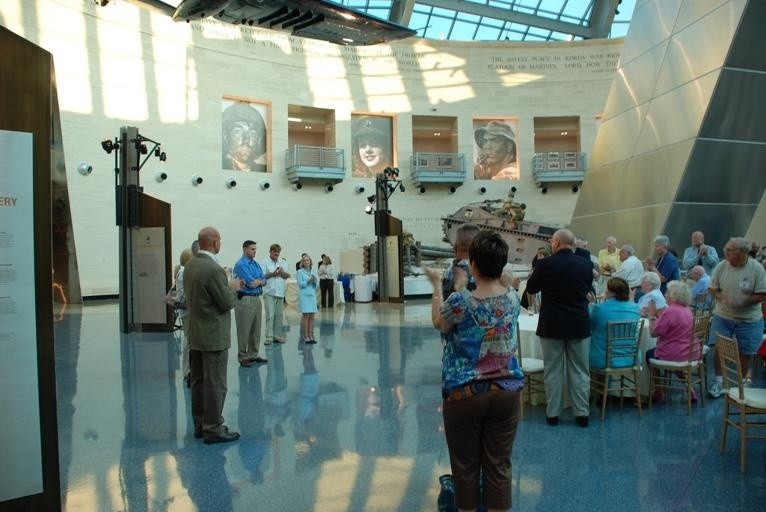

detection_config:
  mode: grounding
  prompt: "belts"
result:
[236,290,259,297]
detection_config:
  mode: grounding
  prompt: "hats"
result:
[222,105,266,155]
[474,119,515,150]
[352,117,392,140]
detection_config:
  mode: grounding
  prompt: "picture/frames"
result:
[222,96,274,173]
[472,115,522,177]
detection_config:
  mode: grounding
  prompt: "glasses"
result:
[723,247,742,252]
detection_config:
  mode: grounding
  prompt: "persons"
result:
[573,236,598,297]
[342,273,351,302]
[182,228,240,444]
[222,105,266,172]
[232,241,268,366]
[191,240,201,256]
[296,256,317,344]
[474,120,518,180]
[537,247,547,260]
[527,229,592,426]
[162,248,193,388]
[586,276,642,413]
[441,224,521,301]
[599,235,621,296]
[263,244,291,344]
[646,280,701,405]
[337,271,344,281]
[318,254,332,269]
[638,272,668,320]
[610,244,645,304]
[422,232,524,512]
[683,230,719,277]
[644,236,680,296]
[689,265,713,312]
[319,256,334,308]
[748,241,760,259]
[707,238,766,398]
[296,253,312,271]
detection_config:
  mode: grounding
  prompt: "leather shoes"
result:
[241,359,253,367]
[194,426,228,437]
[249,357,269,363]
[203,432,240,444]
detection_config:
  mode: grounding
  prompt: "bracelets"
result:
[432,297,443,298]
[649,317,657,320]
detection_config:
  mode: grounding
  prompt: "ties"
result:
[697,248,702,265]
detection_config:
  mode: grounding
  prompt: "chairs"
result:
[515,319,547,421]
[694,288,710,311]
[596,293,606,302]
[648,312,712,418]
[714,331,761,475]
[591,318,647,421]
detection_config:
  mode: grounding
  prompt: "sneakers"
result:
[273,336,286,343]
[545,415,560,426]
[646,391,665,402]
[576,416,590,427]
[264,337,271,345]
[309,336,314,342]
[707,380,726,399]
[304,336,310,343]
[686,392,698,404]
